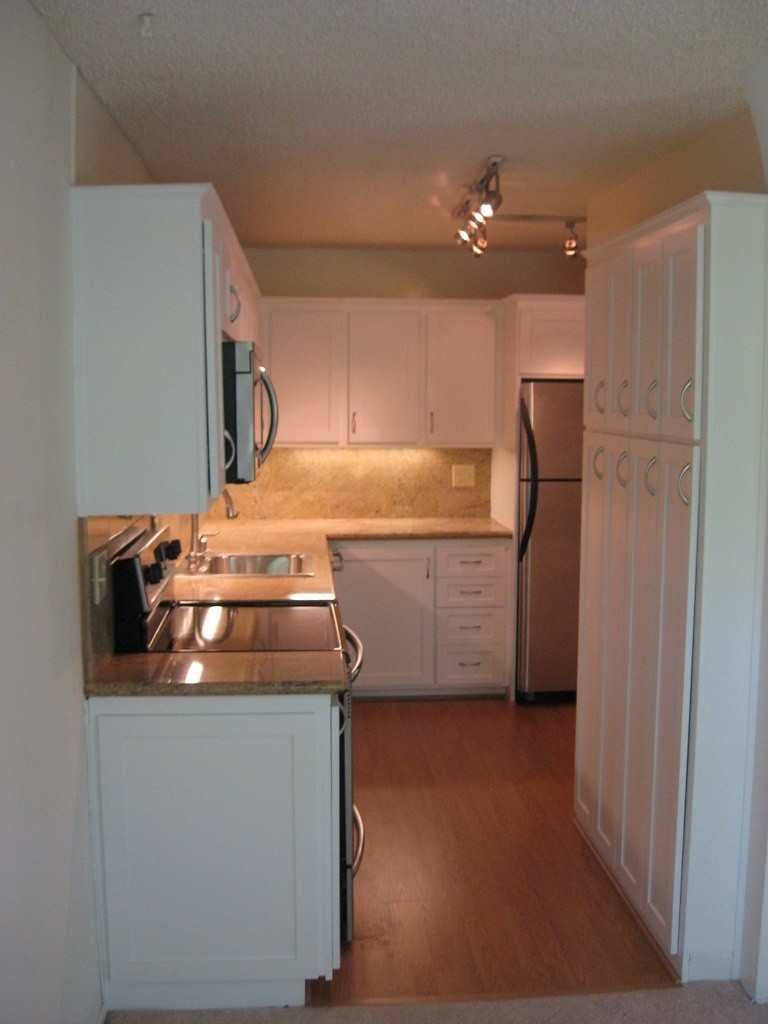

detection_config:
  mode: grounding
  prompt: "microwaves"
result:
[220,339,278,484]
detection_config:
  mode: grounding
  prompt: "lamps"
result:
[564,219,580,257]
[451,157,503,255]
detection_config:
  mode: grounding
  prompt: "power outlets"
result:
[94,551,109,605]
[452,463,476,488]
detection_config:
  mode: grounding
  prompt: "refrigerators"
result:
[519,378,583,706]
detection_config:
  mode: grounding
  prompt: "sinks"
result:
[199,552,296,577]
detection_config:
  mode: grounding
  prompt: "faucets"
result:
[187,489,238,574]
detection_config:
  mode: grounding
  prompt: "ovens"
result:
[337,620,364,941]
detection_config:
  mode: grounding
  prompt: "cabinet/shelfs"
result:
[69,183,263,519]
[256,293,586,448]
[573,189,768,1002]
[331,537,521,701]
[83,696,346,1012]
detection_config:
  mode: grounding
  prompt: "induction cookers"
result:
[146,599,343,651]
